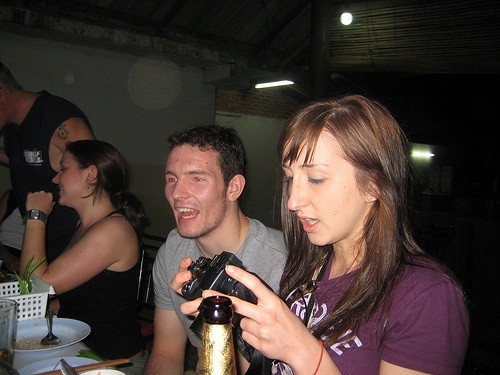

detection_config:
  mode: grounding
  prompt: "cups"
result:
[0,299,17,375]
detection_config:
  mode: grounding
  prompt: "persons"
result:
[145,124,290,375]
[171,95,469,375]
[20,140,145,368]
[0,62,97,273]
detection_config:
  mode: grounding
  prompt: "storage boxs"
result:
[0,275,56,324]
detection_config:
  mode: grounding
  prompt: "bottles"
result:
[198,296,238,375]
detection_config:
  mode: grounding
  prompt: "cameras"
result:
[182,251,273,340]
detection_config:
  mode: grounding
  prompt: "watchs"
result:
[24,209,48,224]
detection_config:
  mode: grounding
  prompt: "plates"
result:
[17,357,105,375]
[0,317,91,353]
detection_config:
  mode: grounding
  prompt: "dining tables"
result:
[12,342,117,371]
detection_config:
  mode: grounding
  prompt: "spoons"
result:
[41,311,61,346]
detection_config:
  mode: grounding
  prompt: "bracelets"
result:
[313,340,323,375]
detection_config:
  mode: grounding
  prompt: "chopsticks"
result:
[36,358,129,375]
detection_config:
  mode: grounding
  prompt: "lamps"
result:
[252,79,295,88]
